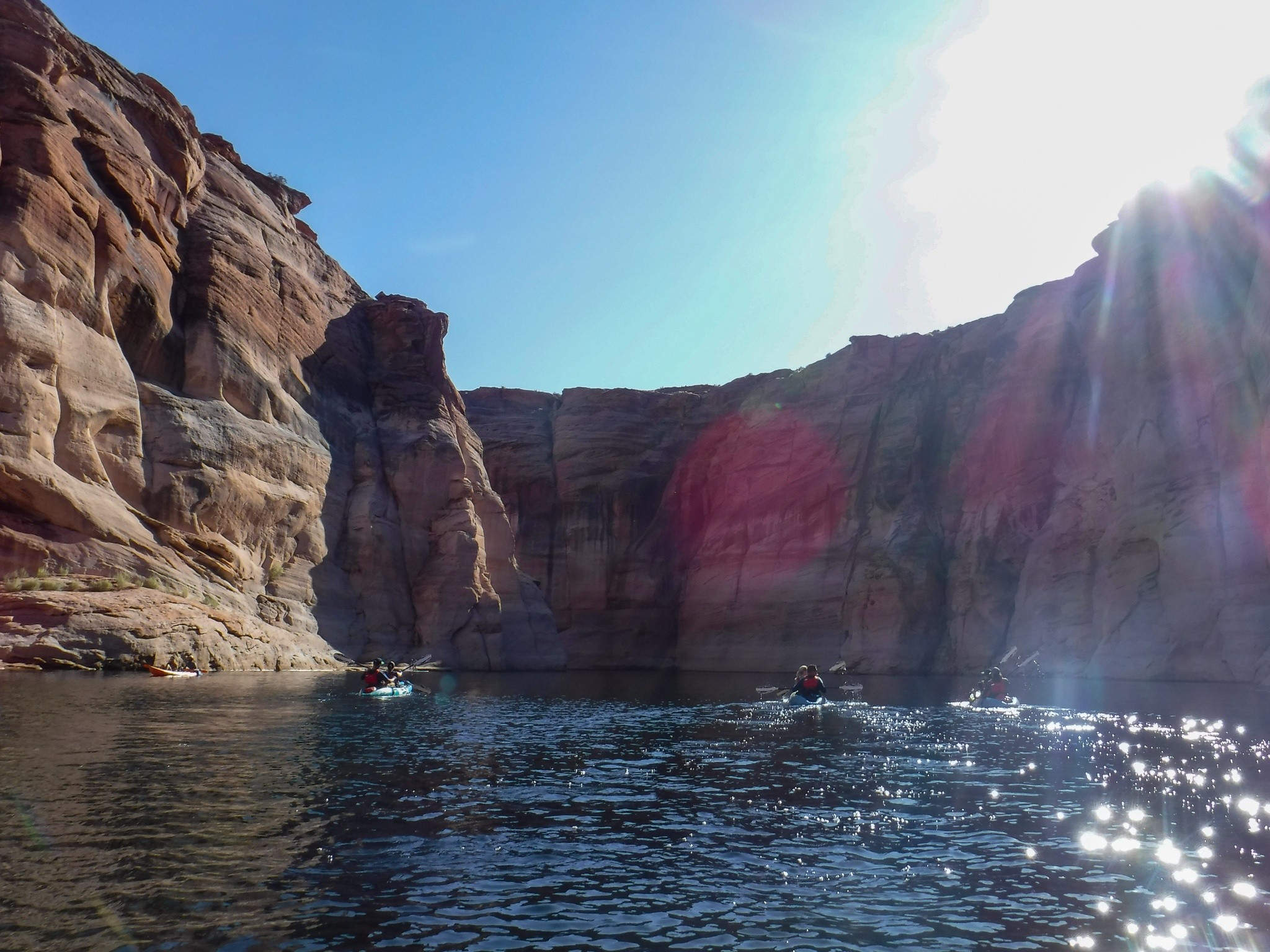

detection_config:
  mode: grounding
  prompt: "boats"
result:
[357,681,413,697]
[142,664,212,678]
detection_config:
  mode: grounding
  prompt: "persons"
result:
[977,666,1010,701]
[783,664,827,699]
[161,655,197,671]
[361,657,403,689]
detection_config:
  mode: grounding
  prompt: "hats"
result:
[374,658,385,664]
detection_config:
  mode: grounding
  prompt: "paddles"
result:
[755,682,864,696]
[967,651,1039,706]
[333,653,431,696]
[143,664,207,674]
[400,653,432,673]
[986,645,1018,680]
[776,659,846,698]
